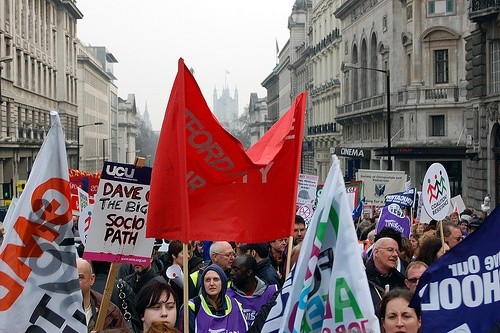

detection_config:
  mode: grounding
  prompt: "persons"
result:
[0,208,500,333]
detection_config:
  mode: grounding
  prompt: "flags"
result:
[0,110,87,333]
[408,205,500,333]
[259,155,381,333]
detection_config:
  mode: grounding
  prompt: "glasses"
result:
[449,237,464,241]
[378,247,400,256]
[407,277,420,283]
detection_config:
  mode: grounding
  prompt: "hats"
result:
[467,219,480,227]
[240,242,269,253]
[461,215,473,223]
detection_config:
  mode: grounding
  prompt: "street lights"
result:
[341,62,393,170]
[77,122,104,170]
